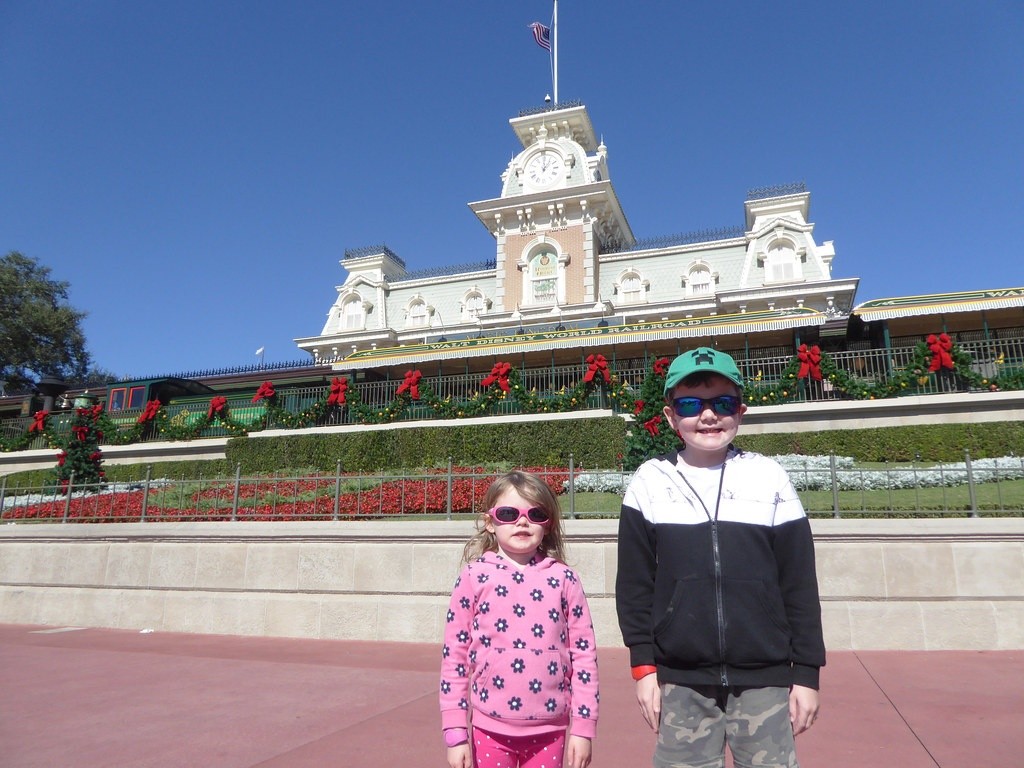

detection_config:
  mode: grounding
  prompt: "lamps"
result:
[475,308,485,339]
[598,293,608,326]
[437,312,447,342]
[556,298,565,331]
[517,302,526,335]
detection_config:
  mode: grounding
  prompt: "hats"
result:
[663,346,745,404]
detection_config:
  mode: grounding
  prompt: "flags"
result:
[527,21,551,52]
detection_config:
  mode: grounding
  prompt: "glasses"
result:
[669,394,742,417]
[487,505,550,524]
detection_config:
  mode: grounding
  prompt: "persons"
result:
[437,470,599,768]
[615,347,827,768]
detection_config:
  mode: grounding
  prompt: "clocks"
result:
[530,154,560,185]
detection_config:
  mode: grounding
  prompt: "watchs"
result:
[631,665,657,681]
[443,729,471,748]
